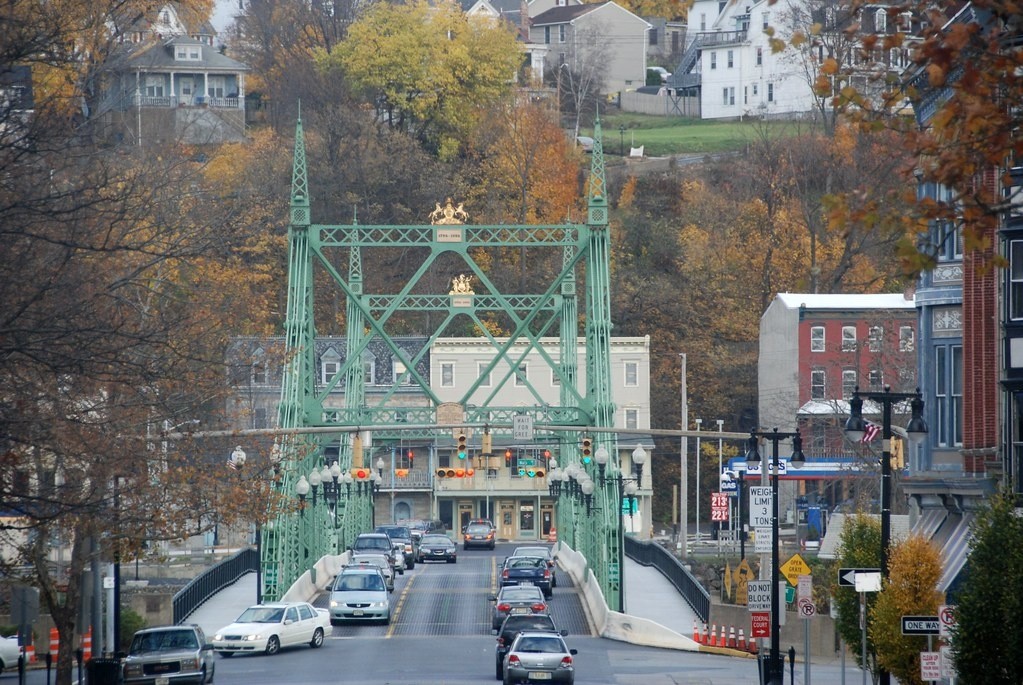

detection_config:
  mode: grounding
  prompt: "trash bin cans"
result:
[84,658,121,685]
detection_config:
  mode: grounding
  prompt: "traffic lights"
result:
[350,468,370,479]
[407,452,414,469]
[505,452,511,468]
[582,439,592,464]
[394,468,409,477]
[457,434,468,462]
[542,451,552,459]
[434,467,474,479]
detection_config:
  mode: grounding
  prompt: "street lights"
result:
[841,383,931,684]
[744,426,806,685]
[231,444,246,514]
[294,457,385,568]
[594,442,648,614]
[543,455,593,517]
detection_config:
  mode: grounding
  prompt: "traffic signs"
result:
[752,613,771,638]
[837,567,894,586]
[901,616,941,635]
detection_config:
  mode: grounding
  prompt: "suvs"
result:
[492,612,568,681]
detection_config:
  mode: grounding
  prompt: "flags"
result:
[859,419,882,444]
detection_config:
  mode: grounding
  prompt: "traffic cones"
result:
[692,619,760,655]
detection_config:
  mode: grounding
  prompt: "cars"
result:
[211,601,334,659]
[501,630,578,685]
[325,516,559,627]
[116,623,216,685]
[487,585,553,635]
[611,66,698,108]
[0,635,30,676]
